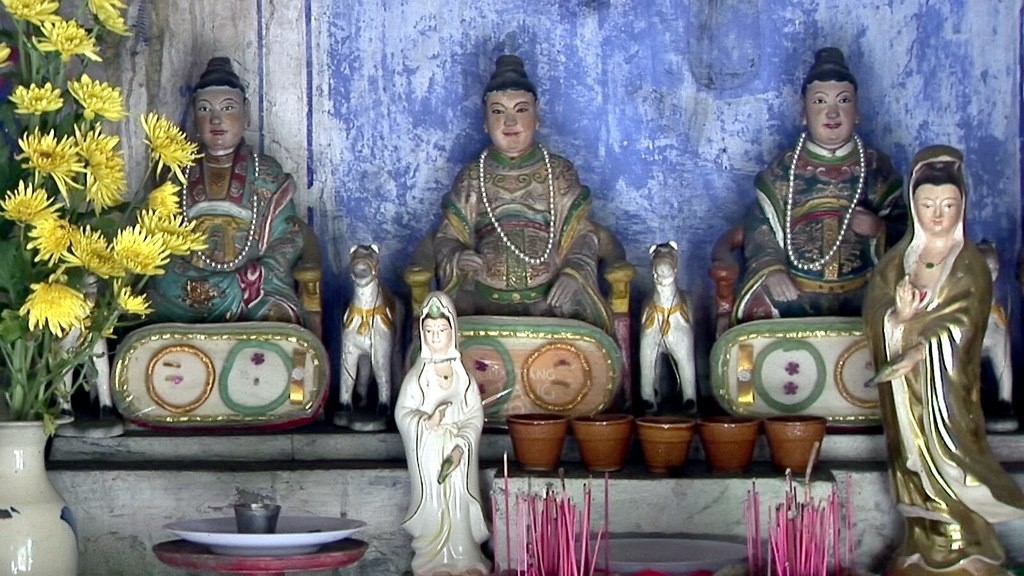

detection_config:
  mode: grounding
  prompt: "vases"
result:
[2,416,79,576]
[508,411,827,476]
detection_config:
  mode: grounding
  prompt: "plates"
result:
[163,517,366,555]
[577,539,754,574]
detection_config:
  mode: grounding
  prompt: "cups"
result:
[570,414,633,472]
[505,413,570,471]
[636,416,695,474]
[695,416,761,477]
[233,503,281,534]
[765,415,827,474]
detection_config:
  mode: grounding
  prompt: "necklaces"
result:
[786,131,865,269]
[919,242,955,268]
[436,364,452,380]
[183,148,258,268]
[478,143,555,264]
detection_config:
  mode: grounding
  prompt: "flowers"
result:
[0,0,206,425]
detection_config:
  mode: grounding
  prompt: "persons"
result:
[727,47,908,327]
[862,144,1024,575]
[394,290,491,576]
[130,58,304,329]
[435,54,613,334]
